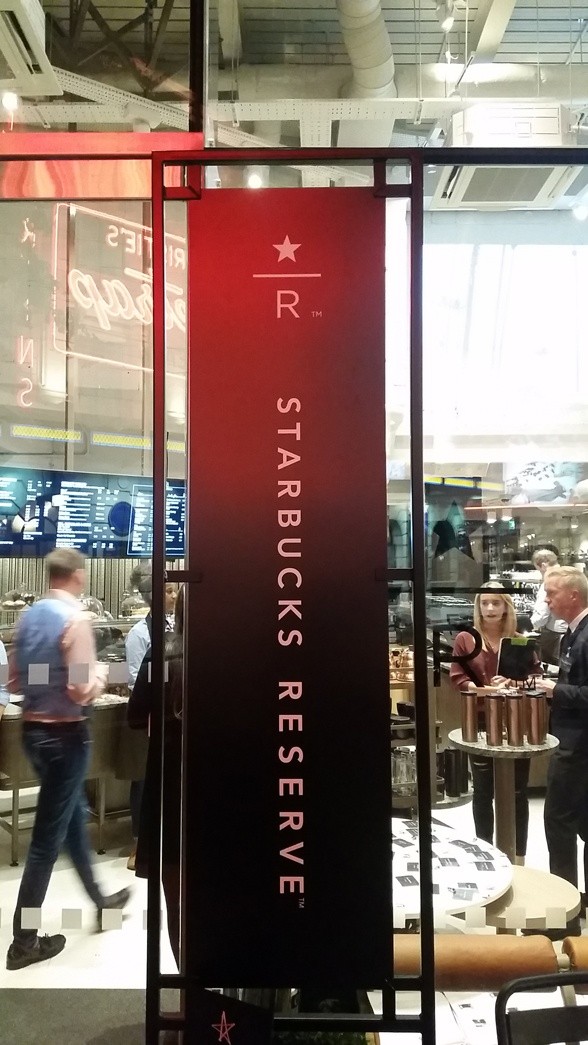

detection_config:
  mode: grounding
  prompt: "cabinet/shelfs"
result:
[1,689,129,867]
[389,647,446,818]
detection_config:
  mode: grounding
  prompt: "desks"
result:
[449,726,559,864]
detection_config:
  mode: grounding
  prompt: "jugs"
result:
[392,747,412,797]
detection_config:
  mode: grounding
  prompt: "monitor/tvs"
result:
[496,636,537,681]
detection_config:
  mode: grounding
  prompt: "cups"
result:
[484,694,503,746]
[506,693,523,746]
[526,692,545,744]
[460,691,478,742]
[539,691,548,739]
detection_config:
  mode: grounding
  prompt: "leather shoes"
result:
[98,888,130,923]
[7,934,66,971]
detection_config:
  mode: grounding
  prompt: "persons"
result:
[129,584,184,973]
[126,573,179,870]
[449,580,545,866]
[530,549,569,666]
[121,563,152,617]
[520,565,588,943]
[0,640,12,719]
[6,546,133,971]
